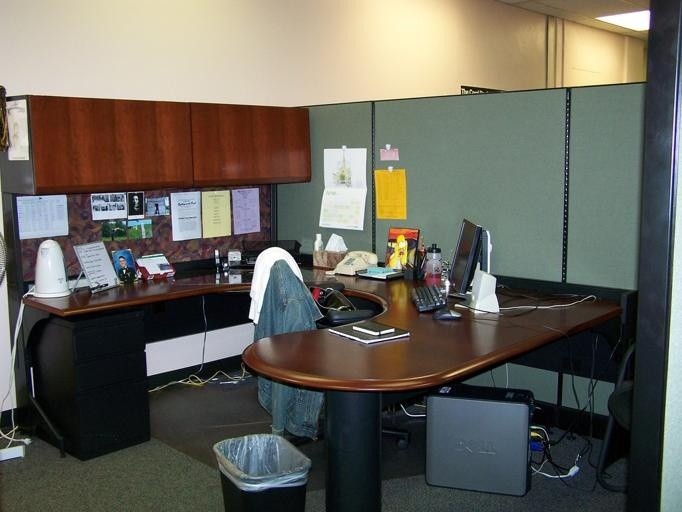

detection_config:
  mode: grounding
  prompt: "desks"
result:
[12,255,626,511]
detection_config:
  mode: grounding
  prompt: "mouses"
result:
[433,309,462,320]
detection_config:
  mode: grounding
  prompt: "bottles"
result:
[426,243,442,287]
[313,233,324,251]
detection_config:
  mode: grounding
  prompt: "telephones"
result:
[336,251,378,276]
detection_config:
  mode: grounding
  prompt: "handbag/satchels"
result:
[307,284,357,330]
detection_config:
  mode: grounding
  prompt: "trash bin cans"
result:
[213,433,312,512]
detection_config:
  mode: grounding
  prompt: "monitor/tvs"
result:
[448,217,492,300]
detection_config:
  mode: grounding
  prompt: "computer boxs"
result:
[426,384,534,496]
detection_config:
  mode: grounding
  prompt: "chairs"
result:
[247,245,414,453]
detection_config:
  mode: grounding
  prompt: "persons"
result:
[130,194,144,215]
[118,255,136,282]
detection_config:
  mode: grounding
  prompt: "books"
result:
[353,320,395,336]
[326,320,412,346]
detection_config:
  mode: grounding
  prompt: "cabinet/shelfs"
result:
[0,94,193,209]
[192,101,315,188]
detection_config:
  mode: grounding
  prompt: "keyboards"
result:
[411,283,447,312]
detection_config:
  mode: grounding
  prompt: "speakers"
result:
[469,269,500,313]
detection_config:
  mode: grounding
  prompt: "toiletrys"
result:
[312,233,324,250]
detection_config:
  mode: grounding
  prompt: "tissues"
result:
[312,234,348,270]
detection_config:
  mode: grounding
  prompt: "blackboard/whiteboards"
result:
[73,240,121,294]
